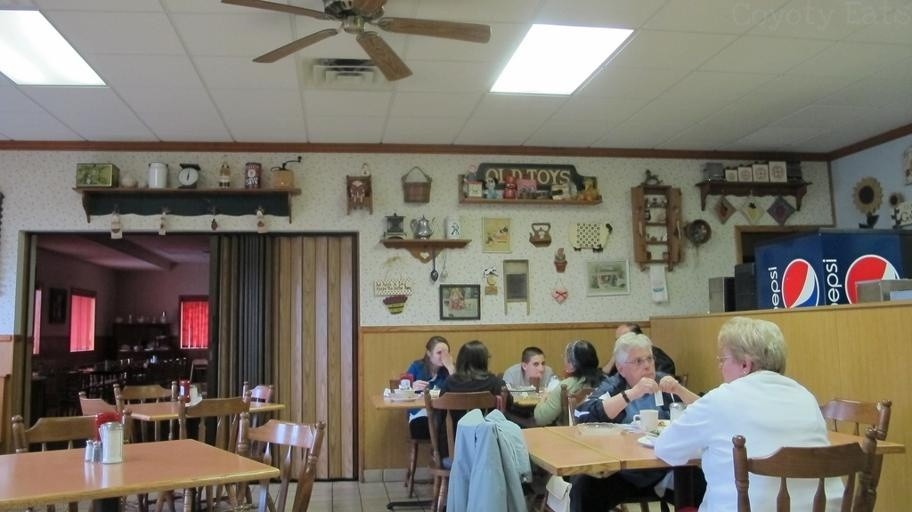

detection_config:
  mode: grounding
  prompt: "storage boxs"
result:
[75,162,120,188]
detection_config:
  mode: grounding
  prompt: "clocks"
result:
[176,162,202,188]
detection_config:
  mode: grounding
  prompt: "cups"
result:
[633,410,659,430]
[668,401,686,425]
[99,419,124,465]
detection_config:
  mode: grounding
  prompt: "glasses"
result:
[623,354,656,367]
[716,355,732,369]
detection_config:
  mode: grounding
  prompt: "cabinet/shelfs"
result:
[625,183,684,271]
[111,319,170,377]
[74,161,305,230]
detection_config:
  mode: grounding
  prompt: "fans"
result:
[219,0,492,86]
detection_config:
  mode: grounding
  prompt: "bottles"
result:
[85,439,102,462]
[218,161,232,190]
[550,374,560,387]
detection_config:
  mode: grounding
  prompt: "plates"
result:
[576,422,623,436]
[654,419,669,429]
[637,435,658,448]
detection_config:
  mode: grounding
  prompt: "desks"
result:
[0,355,912,510]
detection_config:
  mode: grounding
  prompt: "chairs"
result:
[0,355,912,510]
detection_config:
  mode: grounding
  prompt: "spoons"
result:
[431,247,438,282]
[441,248,449,281]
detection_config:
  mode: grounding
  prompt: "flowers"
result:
[886,192,907,230]
[850,174,884,228]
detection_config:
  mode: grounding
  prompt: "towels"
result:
[649,263,670,306]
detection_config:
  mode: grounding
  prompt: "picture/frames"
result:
[50,286,68,327]
[439,282,481,320]
[585,257,633,300]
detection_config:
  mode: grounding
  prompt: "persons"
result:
[654,316,845,512]
[534,339,607,428]
[439,340,513,470]
[504,347,554,392]
[408,336,454,439]
[569,333,702,512]
[599,323,676,376]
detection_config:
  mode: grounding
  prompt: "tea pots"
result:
[528,221,553,247]
[411,214,436,239]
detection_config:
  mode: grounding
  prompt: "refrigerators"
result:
[754,229,912,310]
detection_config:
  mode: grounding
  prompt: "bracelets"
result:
[619,391,630,403]
